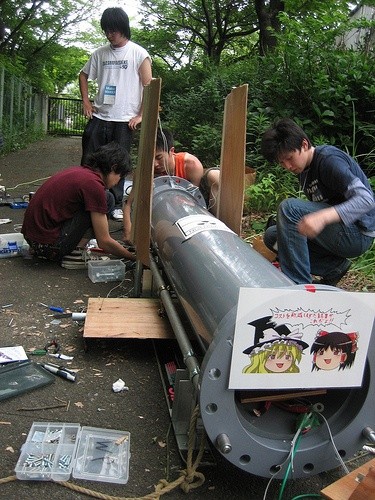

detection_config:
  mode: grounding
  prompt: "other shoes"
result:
[110,208,123,222]
[312,260,352,286]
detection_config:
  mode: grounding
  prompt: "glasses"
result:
[102,31,117,36]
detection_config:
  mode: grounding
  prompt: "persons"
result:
[260,119,375,285]
[21,142,136,270]
[124,129,221,245]
[79,7,152,222]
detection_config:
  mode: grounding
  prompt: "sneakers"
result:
[61,247,110,270]
